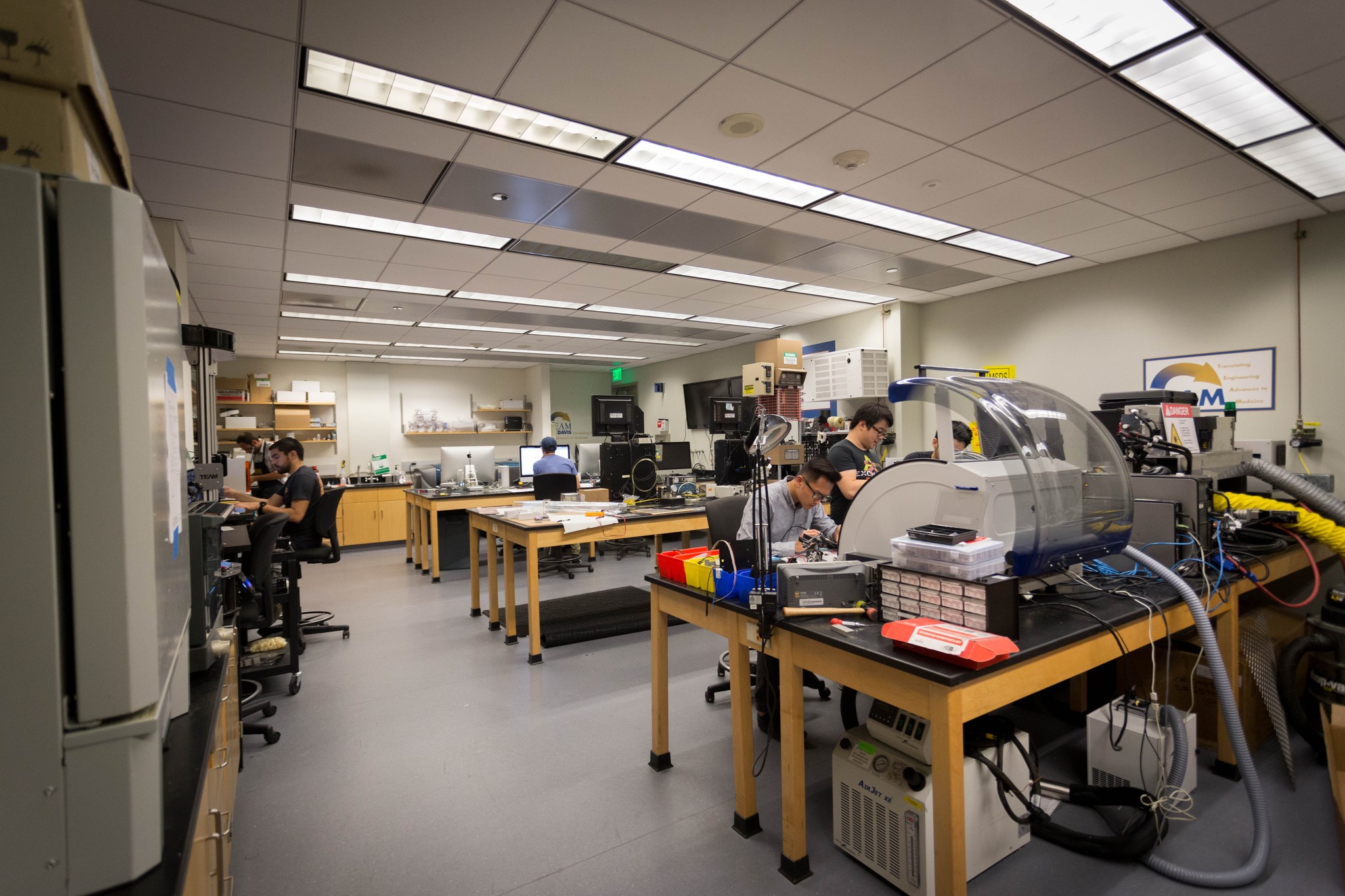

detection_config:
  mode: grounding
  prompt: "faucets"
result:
[367,462,373,482]
[357,465,362,484]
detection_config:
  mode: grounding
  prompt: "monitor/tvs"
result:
[440,446,495,483]
[591,395,635,436]
[519,444,571,483]
[655,442,692,475]
[578,443,601,477]
[709,397,744,435]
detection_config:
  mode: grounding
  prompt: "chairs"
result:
[213,508,288,768]
[243,484,351,655]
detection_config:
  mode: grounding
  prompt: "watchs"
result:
[257,501,267,516]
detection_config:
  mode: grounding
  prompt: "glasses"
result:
[803,477,832,502]
[871,425,887,438]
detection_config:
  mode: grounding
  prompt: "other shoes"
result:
[756,712,807,743]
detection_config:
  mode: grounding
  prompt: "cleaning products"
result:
[340,461,346,485]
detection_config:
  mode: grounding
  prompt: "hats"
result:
[540,437,558,451]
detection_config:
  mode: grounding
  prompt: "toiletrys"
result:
[394,464,399,482]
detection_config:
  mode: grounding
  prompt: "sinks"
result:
[351,480,396,486]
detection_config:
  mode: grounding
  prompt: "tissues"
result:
[381,474,396,482]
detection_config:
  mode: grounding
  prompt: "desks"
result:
[643,524,1342,895]
[403,488,612,585]
[466,500,835,666]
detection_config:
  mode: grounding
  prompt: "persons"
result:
[735,454,844,557]
[222,437,325,549]
[829,402,894,524]
[903,421,972,463]
[533,438,582,564]
[236,432,289,497]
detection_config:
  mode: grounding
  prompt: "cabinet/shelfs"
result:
[344,486,412,549]
[216,398,338,454]
[471,394,534,434]
[181,631,245,896]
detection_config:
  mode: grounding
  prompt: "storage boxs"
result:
[225,416,256,429]
[306,391,335,403]
[276,392,306,402]
[216,372,271,402]
[755,338,803,370]
[292,380,320,392]
[274,405,311,430]
[499,400,524,409]
